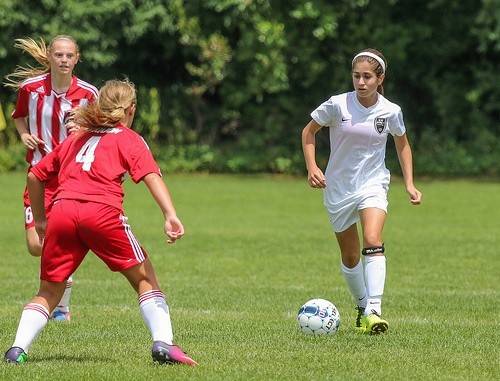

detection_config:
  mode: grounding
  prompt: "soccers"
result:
[297,298,340,336]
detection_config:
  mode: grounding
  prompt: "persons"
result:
[302,48,423,335]
[3,35,100,322]
[4,77,201,369]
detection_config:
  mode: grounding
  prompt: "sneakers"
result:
[363,309,389,337]
[51,311,70,321]
[151,341,200,367]
[4,347,28,363]
[354,304,366,334]
